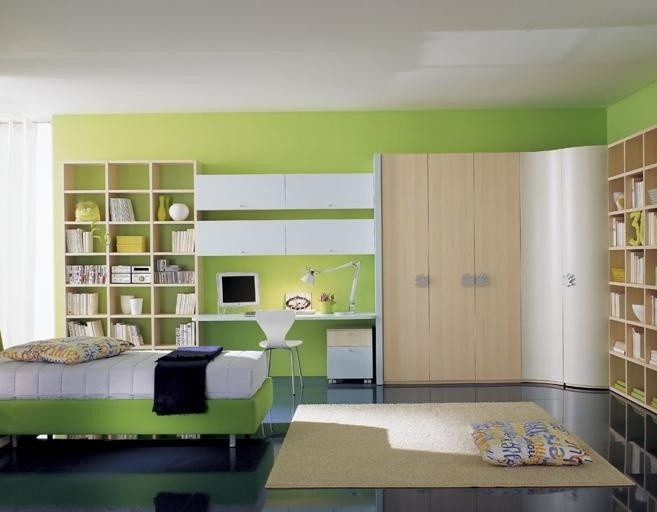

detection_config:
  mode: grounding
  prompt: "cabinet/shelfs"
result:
[60,159,203,348]
[520,142,607,392]
[431,385,521,511]
[325,326,374,384]
[606,125,656,414]
[428,152,521,387]
[194,172,375,213]
[377,385,431,512]
[520,382,608,511]
[194,218,375,255]
[608,392,656,511]
[374,153,428,387]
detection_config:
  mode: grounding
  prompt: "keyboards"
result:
[244,311,257,317]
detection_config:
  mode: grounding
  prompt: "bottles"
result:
[156,195,169,221]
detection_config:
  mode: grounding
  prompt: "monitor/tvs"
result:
[219,272,260,308]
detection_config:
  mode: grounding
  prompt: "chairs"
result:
[255,309,305,397]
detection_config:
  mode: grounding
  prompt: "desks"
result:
[193,310,375,322]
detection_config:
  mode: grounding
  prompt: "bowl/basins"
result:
[634,487,647,502]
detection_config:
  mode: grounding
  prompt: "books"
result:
[609,175,657,409]
[610,387,657,512]
[65,197,196,349]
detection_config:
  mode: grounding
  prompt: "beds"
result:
[0,439,272,511]
[0,349,273,449]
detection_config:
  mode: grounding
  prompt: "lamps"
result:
[299,258,361,311]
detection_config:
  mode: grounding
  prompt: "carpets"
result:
[265,399,638,490]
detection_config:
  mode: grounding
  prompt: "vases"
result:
[164,197,170,220]
[156,195,166,221]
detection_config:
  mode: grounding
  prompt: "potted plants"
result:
[317,290,336,315]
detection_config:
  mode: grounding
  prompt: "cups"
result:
[120,295,134,315]
[129,298,142,315]
[631,303,645,323]
[611,192,623,210]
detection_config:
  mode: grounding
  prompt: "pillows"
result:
[1,334,104,362]
[469,419,592,466]
[38,335,135,364]
